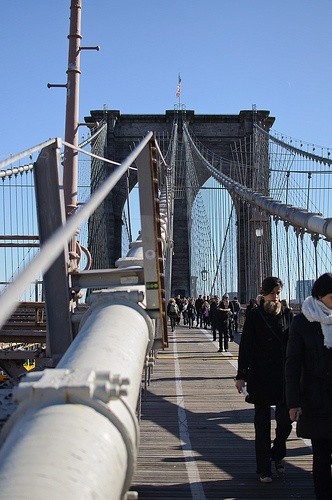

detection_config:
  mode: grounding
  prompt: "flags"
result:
[176,72,182,96]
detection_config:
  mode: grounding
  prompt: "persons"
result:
[167,294,293,353]
[285,273,332,500]
[235,277,295,483]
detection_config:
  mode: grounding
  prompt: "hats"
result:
[222,293,229,299]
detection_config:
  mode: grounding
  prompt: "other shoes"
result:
[217,349,223,352]
[230,340,234,342]
[225,349,228,352]
[213,339,216,341]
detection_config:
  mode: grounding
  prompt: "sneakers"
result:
[258,474,272,483]
[274,459,286,475]
